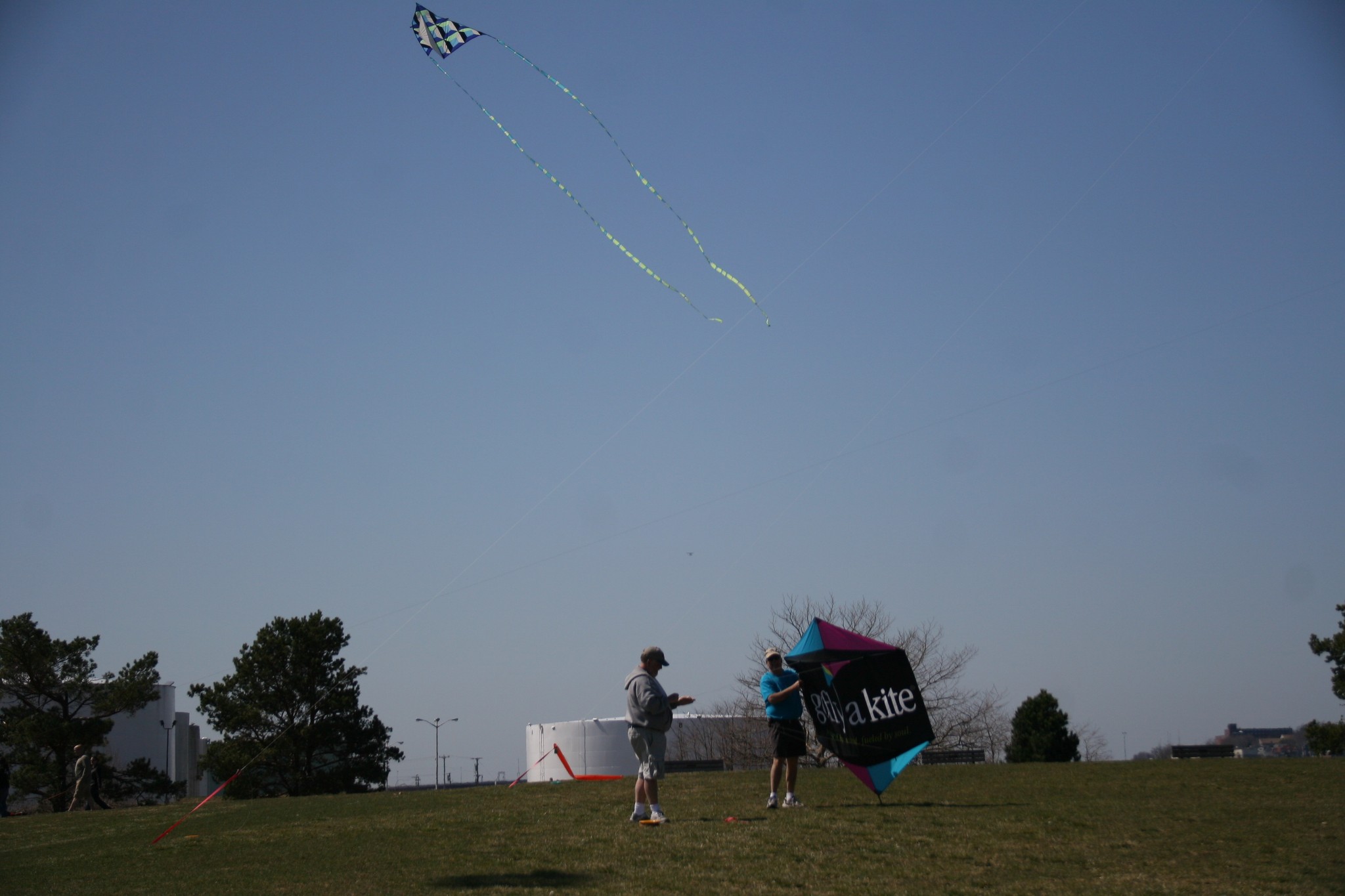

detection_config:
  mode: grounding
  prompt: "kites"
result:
[783,617,937,804]
[409,3,770,332]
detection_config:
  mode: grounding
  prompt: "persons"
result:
[760,648,807,809]
[624,647,696,823]
[69,745,113,813]
[1233,743,1331,760]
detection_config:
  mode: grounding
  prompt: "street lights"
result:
[160,719,177,805]
[415,717,458,790]
[385,740,404,792]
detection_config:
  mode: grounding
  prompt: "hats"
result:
[643,646,669,666]
[765,647,780,660]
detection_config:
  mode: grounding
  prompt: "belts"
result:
[628,724,637,728]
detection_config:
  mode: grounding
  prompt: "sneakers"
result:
[650,811,670,823]
[630,812,649,822]
[782,797,805,807]
[767,795,778,808]
